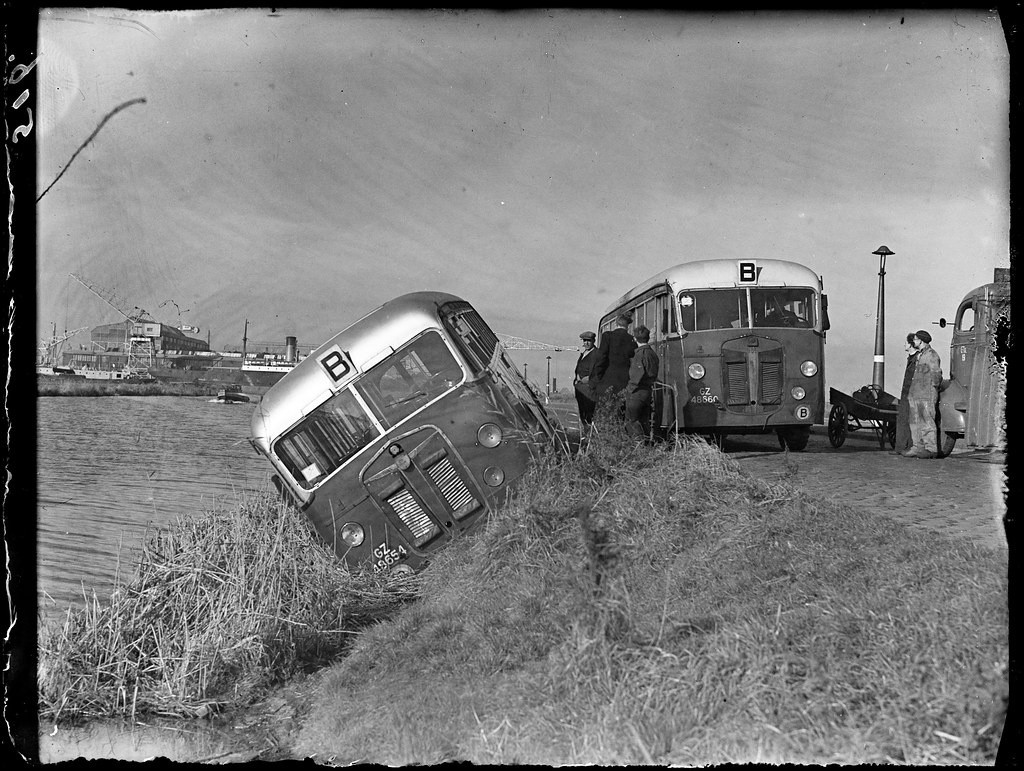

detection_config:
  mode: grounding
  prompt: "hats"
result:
[916,331,931,343]
[580,331,596,341]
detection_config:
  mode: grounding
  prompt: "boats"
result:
[217,385,250,404]
[241,334,302,386]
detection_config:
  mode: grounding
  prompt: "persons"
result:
[760,294,799,327]
[572,310,659,458]
[889,330,943,459]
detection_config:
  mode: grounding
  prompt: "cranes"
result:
[492,332,580,353]
[37,271,164,380]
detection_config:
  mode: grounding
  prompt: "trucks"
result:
[935,268,1011,458]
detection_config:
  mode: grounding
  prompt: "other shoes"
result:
[903,446,917,457]
[889,450,899,454]
[917,450,938,459]
[900,446,911,455]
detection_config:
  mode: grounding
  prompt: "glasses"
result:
[913,338,919,341]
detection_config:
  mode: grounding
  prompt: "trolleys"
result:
[830,387,901,453]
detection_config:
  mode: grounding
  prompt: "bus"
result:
[245,292,562,601]
[598,260,829,451]
